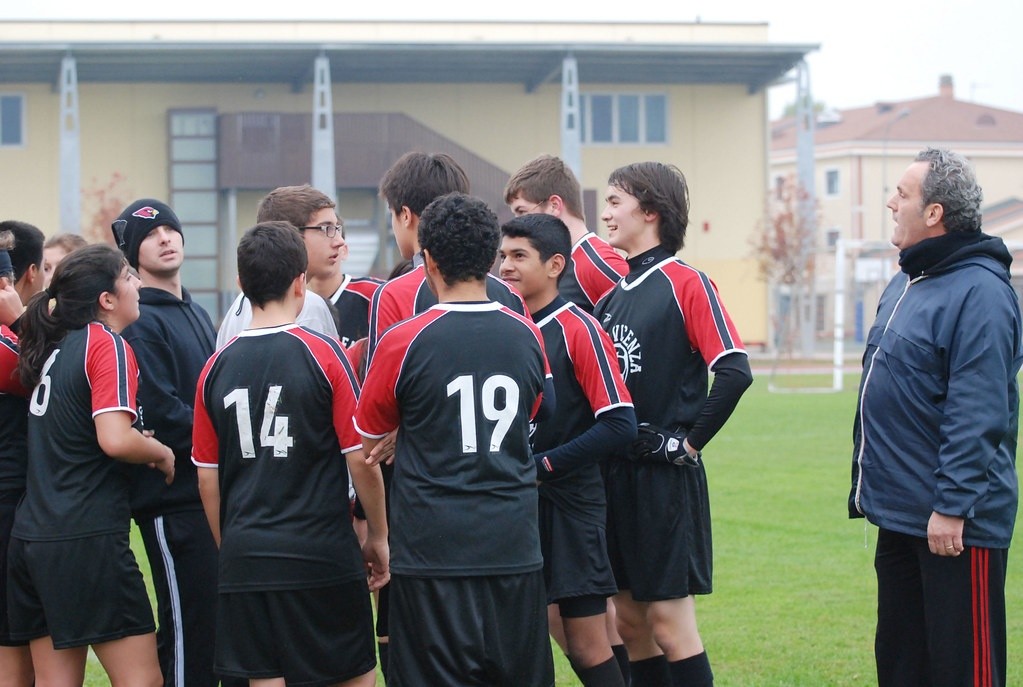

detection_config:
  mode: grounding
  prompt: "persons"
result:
[848,149,1023,686]
[0,152,753,687]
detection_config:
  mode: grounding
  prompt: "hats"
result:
[111,198,184,273]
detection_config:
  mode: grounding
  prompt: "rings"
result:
[945,546,956,549]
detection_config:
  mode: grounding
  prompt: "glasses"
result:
[299,225,343,238]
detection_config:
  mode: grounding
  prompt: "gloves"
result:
[632,422,702,467]
